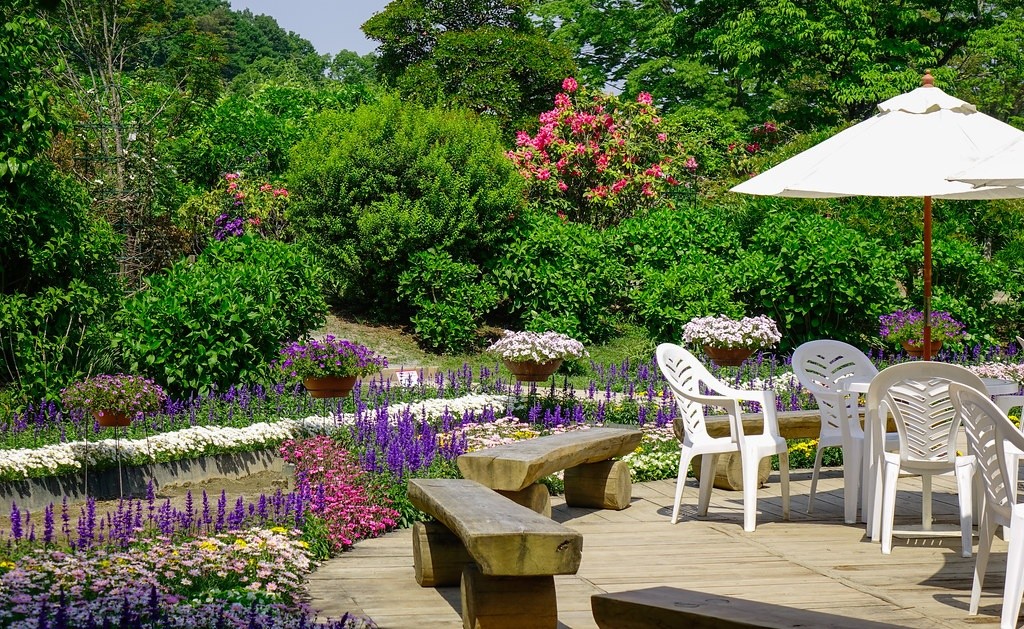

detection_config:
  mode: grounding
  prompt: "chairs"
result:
[656,342,791,532]
[861,361,1024,628]
[792,340,897,524]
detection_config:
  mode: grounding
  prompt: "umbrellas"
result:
[726,68,1024,360]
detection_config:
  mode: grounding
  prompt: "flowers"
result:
[879,310,965,345]
[486,329,590,362]
[682,314,784,348]
[269,333,390,381]
[62,373,165,419]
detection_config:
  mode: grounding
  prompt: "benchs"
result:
[457,427,644,520]
[592,587,916,629]
[407,479,583,629]
[672,406,898,492]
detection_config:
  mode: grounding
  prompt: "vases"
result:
[506,359,562,381]
[90,410,134,426]
[703,346,755,366]
[303,375,355,397]
[902,342,942,357]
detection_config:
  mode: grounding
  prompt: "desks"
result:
[847,378,1018,538]
